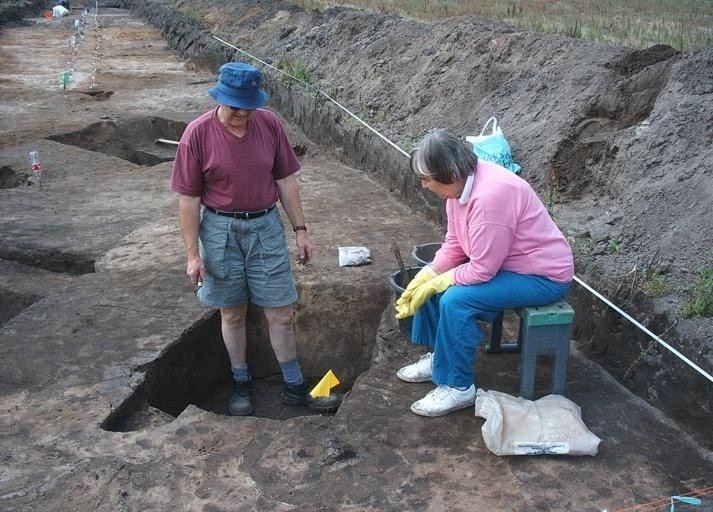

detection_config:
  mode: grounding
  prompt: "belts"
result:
[204,205,277,220]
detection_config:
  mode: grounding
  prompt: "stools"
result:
[484,297,576,400]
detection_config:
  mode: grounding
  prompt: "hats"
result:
[208,61,270,110]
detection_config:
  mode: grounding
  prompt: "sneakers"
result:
[396,351,434,384]
[410,383,476,418]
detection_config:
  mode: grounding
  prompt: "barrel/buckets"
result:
[412,242,443,265]
[389,266,431,341]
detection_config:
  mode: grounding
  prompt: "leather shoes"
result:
[229,372,254,416]
[280,377,341,413]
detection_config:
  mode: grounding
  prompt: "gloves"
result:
[395,265,457,320]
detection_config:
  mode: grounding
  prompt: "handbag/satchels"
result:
[464,116,522,176]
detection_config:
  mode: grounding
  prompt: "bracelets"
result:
[293,226,306,231]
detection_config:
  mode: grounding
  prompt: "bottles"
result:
[28,150,41,179]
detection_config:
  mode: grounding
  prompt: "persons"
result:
[170,62,340,417]
[395,128,575,417]
